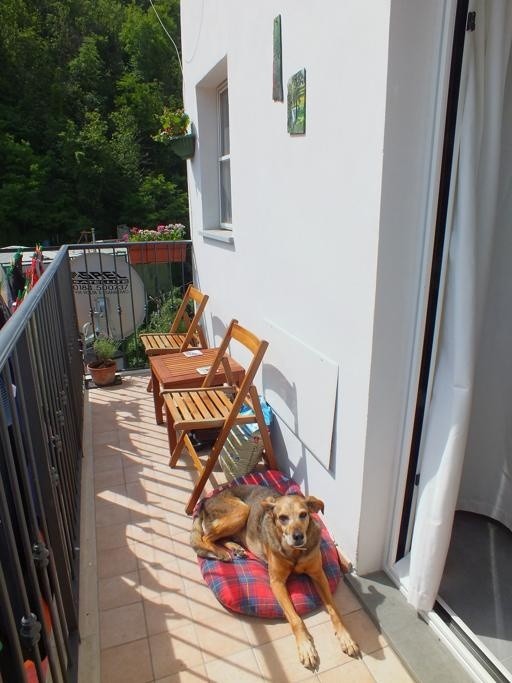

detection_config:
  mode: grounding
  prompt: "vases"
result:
[126,245,188,266]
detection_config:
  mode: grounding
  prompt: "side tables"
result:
[148,347,245,457]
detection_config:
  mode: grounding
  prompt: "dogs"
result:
[189,484,362,672]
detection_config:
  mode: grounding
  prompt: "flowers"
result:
[121,222,188,242]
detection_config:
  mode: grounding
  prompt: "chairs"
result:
[158,318,279,516]
[138,282,210,393]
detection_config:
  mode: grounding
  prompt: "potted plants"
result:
[148,105,194,161]
[86,338,118,386]
